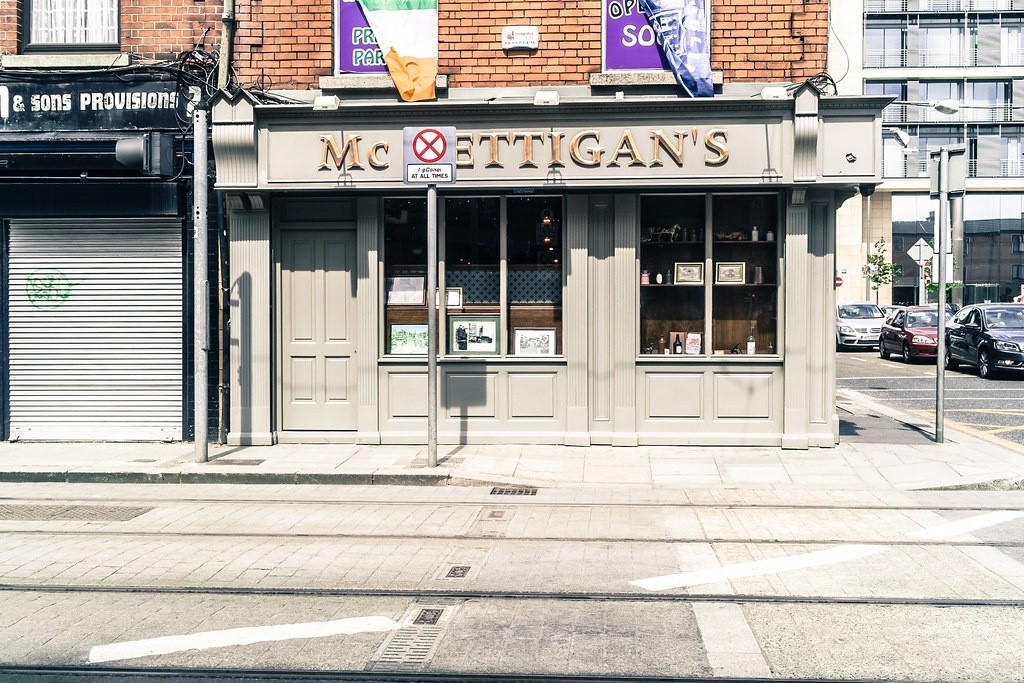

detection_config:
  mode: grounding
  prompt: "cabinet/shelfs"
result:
[639,238,777,287]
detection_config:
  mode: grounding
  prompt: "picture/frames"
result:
[390,323,428,355]
[716,262,745,284]
[426,287,462,309]
[674,262,704,285]
[446,313,501,356]
[513,327,557,356]
[384,274,426,306]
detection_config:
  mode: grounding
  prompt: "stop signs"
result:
[835,276,843,287]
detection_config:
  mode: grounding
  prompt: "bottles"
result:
[682,227,704,242]
[640,270,650,284]
[665,269,672,284]
[658,335,666,354]
[746,326,756,355]
[766,224,774,241]
[656,263,663,284]
[768,342,774,354]
[673,334,682,354]
[755,263,764,283]
[751,226,758,241]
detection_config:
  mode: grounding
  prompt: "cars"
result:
[944,302,1024,381]
[836,301,887,352]
[879,302,960,364]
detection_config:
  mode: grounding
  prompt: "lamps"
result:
[534,91,560,105]
[892,100,958,114]
[313,94,340,111]
[890,127,910,146]
